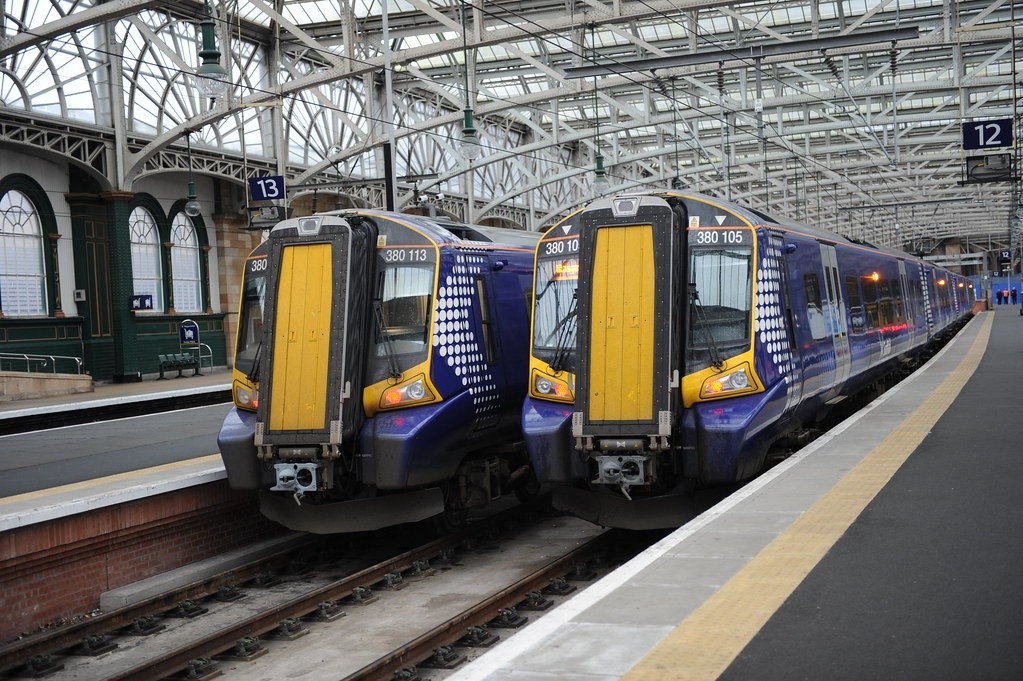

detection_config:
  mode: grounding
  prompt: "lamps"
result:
[182,128,201,216]
[587,21,608,194]
[195,0,230,96]
[458,0,481,160]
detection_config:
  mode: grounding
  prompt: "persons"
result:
[996,289,1002,305]
[1010,287,1017,304]
[1002,289,1009,304]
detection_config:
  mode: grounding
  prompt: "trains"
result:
[847,296,922,332]
[519,185,973,530]
[217,191,544,539]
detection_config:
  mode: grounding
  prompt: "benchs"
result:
[158,352,205,381]
